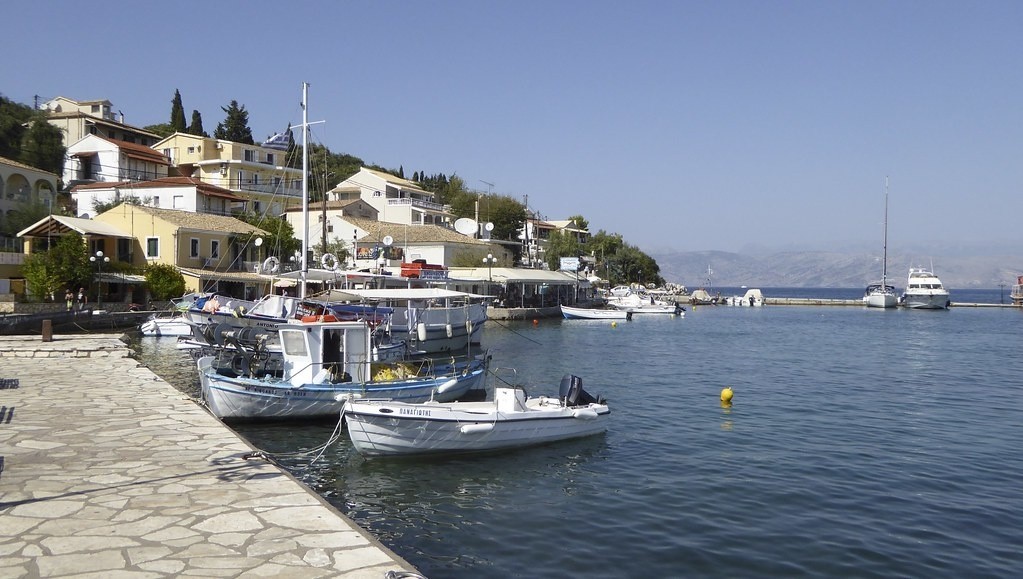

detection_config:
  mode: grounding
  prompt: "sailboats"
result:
[861,174,900,309]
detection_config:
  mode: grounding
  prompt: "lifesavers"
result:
[367,316,381,329]
[321,252,339,271]
[263,256,280,273]
[300,314,336,322]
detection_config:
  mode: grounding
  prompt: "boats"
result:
[900,255,951,310]
[602,290,687,316]
[560,303,634,321]
[330,364,611,459]
[688,288,716,305]
[1009,274,1023,305]
[135,82,500,426]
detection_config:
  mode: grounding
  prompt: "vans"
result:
[604,286,629,296]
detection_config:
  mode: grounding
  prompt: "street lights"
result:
[584,266,589,301]
[481,254,499,301]
[89,250,111,309]
[290,252,303,299]
[575,261,581,304]
[637,269,642,294]
